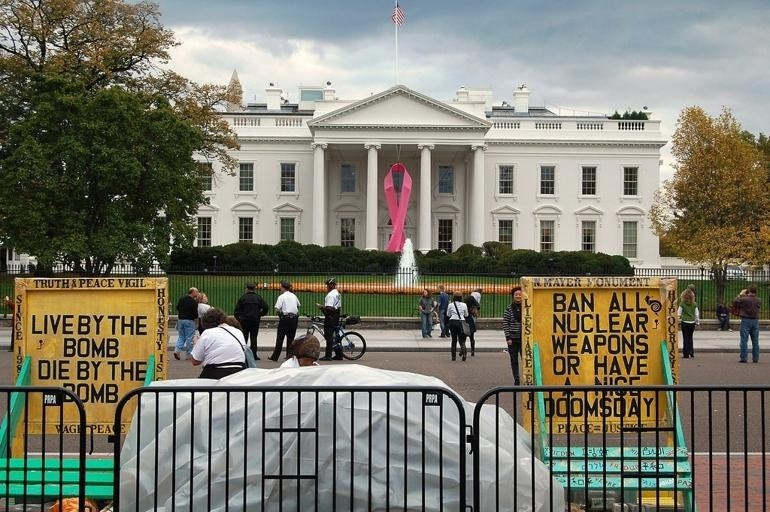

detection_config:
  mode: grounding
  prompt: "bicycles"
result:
[301,312,367,360]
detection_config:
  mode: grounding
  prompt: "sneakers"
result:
[254,356,260,360]
[268,356,278,361]
[332,356,343,360]
[439,333,450,337]
[319,356,332,360]
[422,333,432,338]
[174,351,180,360]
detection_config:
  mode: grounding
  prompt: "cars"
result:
[709,263,744,281]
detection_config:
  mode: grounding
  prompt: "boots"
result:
[451,341,475,361]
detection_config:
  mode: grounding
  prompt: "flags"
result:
[391,4,406,28]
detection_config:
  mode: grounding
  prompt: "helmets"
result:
[325,278,336,284]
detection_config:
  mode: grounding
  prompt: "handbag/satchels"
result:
[459,320,472,336]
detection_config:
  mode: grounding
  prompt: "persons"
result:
[3,298,15,352]
[172,277,342,380]
[687,283,695,292]
[466,288,483,339]
[733,284,762,363]
[437,285,451,338]
[502,287,522,386]
[677,288,700,359]
[447,291,469,362]
[715,304,733,332]
[418,288,436,338]
[458,295,481,357]
